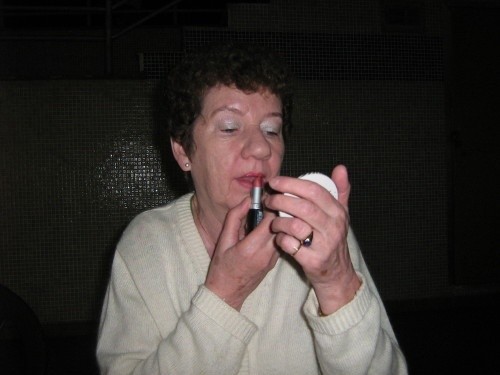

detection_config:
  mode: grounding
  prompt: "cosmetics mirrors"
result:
[279,171,339,218]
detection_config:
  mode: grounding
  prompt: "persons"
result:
[97,42,408,375]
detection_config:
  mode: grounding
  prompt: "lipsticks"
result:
[245,175,265,238]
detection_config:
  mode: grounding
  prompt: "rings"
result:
[292,239,302,256]
[303,231,313,247]
